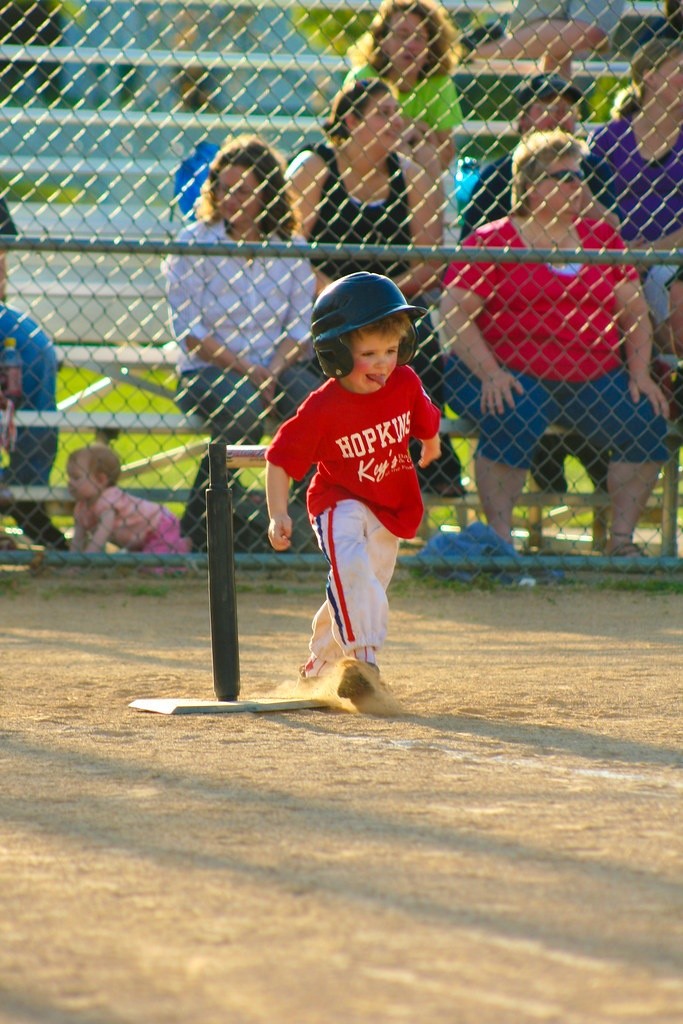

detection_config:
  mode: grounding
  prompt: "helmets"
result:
[311,271,427,382]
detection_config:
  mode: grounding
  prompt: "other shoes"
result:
[294,667,325,694]
[337,660,394,718]
[599,544,649,557]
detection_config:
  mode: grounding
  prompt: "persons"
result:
[338,0,466,172]
[1,190,69,551]
[67,445,194,577]
[473,0,625,59]
[264,270,442,708]
[583,38,683,394]
[441,129,674,559]
[280,79,466,495]
[161,135,325,556]
[456,70,620,495]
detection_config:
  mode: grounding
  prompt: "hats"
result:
[520,71,587,103]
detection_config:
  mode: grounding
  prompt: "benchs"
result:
[1,0,682,558]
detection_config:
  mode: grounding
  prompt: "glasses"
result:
[541,169,585,183]
